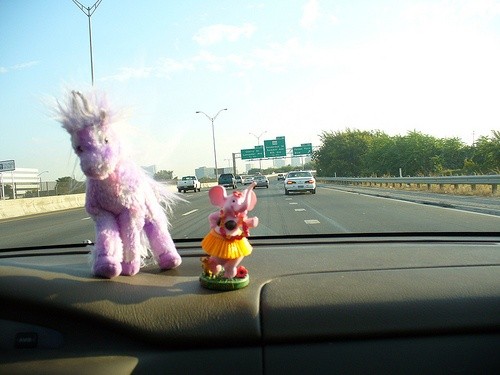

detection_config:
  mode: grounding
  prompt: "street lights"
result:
[249,131,267,173]
[37,170,49,191]
[195,108,228,182]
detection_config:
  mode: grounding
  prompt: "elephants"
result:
[200,181,259,277]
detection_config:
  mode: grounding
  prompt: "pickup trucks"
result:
[177,175,201,193]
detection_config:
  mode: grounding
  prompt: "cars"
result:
[276,173,285,181]
[283,170,317,195]
[234,172,262,186]
[251,175,270,189]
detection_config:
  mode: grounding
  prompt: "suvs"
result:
[217,173,238,189]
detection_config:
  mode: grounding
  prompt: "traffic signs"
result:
[293,143,312,155]
[240,145,265,159]
[263,135,287,157]
